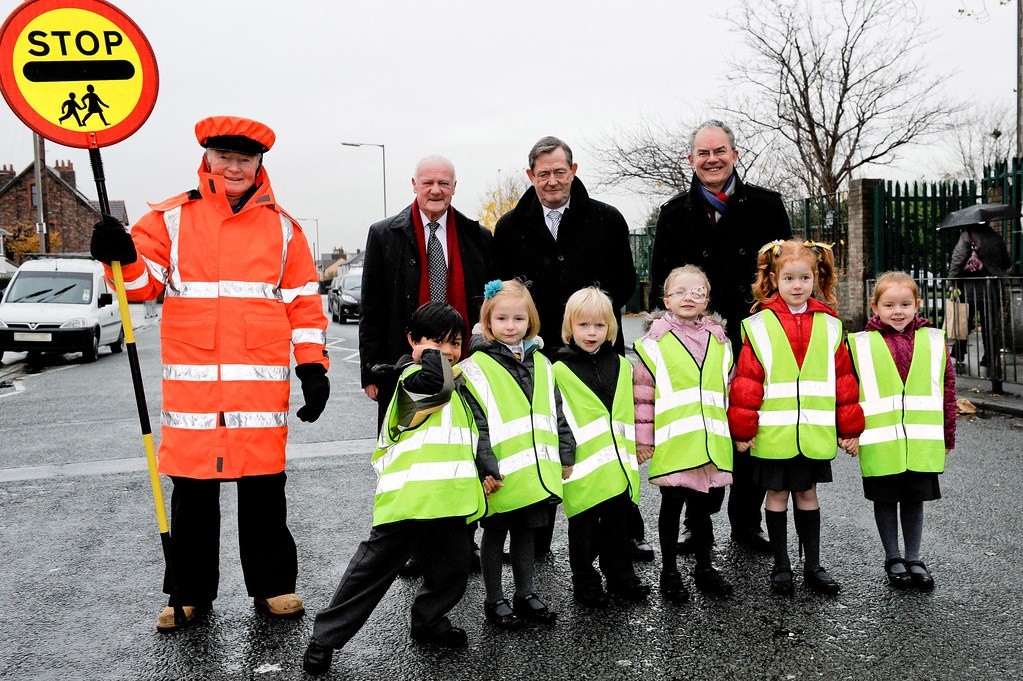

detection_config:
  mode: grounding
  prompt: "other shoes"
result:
[511,592,557,625]
[576,591,610,611]
[804,564,840,592]
[604,585,649,607]
[695,568,725,596]
[659,573,690,599]
[400,542,510,576]
[485,599,517,625]
[884,556,912,588]
[407,625,468,645]
[303,640,334,672]
[906,560,935,592]
[252,594,306,617]
[155,602,215,632]
[771,564,795,594]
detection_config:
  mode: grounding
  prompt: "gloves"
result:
[293,364,331,423]
[92,219,137,267]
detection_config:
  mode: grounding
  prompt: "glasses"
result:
[667,286,709,305]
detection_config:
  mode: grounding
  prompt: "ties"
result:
[548,211,562,241]
[427,223,447,304]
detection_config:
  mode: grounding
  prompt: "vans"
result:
[0,253,124,361]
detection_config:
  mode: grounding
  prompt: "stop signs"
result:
[0,0,160,147]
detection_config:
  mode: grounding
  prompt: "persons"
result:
[494,137,655,568]
[728,239,866,596]
[649,120,800,556]
[90,115,331,634]
[837,270,956,590]
[302,300,505,675]
[631,264,756,603]
[452,277,577,628]
[550,285,654,608]
[359,154,497,579]
[945,221,1013,378]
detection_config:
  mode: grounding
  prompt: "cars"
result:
[325,276,362,324]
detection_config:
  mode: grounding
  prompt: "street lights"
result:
[300,218,319,270]
[341,142,386,221]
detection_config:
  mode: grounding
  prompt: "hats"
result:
[194,117,276,156]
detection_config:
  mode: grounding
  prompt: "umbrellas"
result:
[934,202,1023,228]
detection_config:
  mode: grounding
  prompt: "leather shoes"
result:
[631,540,655,563]
[731,531,771,554]
[676,530,693,552]
